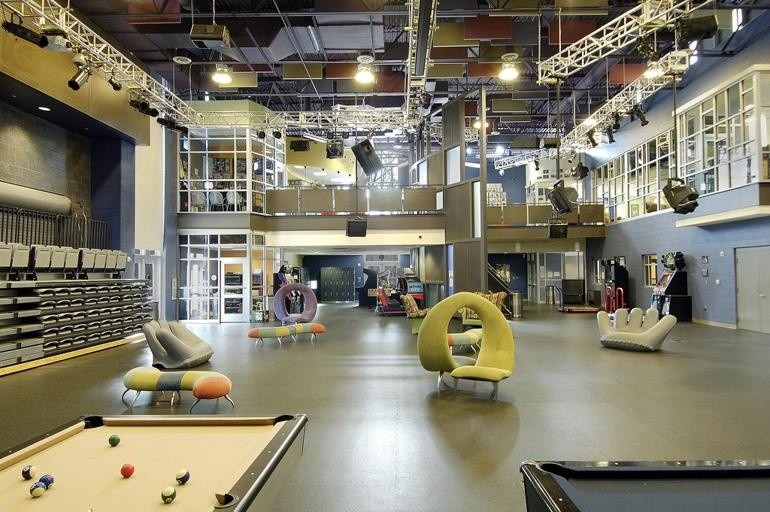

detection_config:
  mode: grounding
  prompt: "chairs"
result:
[397,289,515,404]
[273,281,318,326]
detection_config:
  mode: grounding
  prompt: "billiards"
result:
[40,474,53,489]
[22,465,36,479]
[30,482,46,497]
[161,487,176,503]
[176,470,190,484]
[121,464,134,478]
[110,435,120,447]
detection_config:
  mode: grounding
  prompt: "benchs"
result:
[246,323,326,347]
[121,367,236,414]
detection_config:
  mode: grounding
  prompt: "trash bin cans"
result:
[512,292,523,318]
[545,286,555,305]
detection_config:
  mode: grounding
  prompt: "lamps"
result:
[0,10,236,140]
[470,16,721,151]
[251,53,392,182]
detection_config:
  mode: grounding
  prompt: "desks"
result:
[521,457,769,512]
[0,412,309,512]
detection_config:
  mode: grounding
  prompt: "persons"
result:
[275,266,293,317]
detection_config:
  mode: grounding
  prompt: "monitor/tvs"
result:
[575,162,589,179]
[547,180,578,216]
[663,179,699,214]
[290,140,310,152]
[253,160,259,172]
[654,271,673,292]
[293,275,299,279]
[310,279,318,290]
[325,140,345,158]
[548,223,568,239]
[183,135,188,150]
[346,219,366,237]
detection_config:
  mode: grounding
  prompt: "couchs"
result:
[597,306,677,354]
[140,320,214,368]
